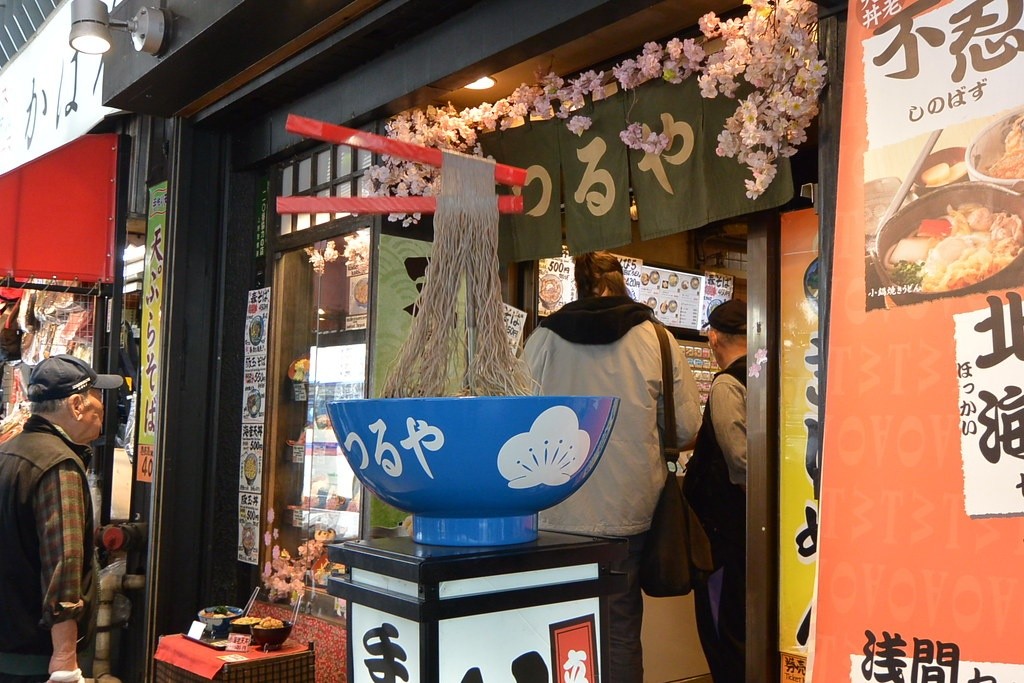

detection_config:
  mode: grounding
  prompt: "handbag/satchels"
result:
[629,321,714,598]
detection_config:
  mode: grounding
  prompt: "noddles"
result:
[376,148,545,398]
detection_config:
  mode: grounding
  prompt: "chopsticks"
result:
[288,596,304,639]
[243,586,260,618]
[873,129,944,236]
[274,112,527,215]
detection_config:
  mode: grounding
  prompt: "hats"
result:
[28,354,124,401]
[702,298,747,334]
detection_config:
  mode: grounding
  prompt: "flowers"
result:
[363,1,827,230]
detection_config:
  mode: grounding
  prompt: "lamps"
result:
[69,0,167,56]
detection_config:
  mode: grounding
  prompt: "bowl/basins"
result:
[242,522,255,557]
[326,396,621,547]
[198,606,293,649]
[872,110,1024,306]
[250,315,263,346]
[244,453,258,486]
[247,389,261,417]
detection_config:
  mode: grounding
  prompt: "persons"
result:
[516,251,702,683]
[683,299,746,683]
[0,355,124,683]
